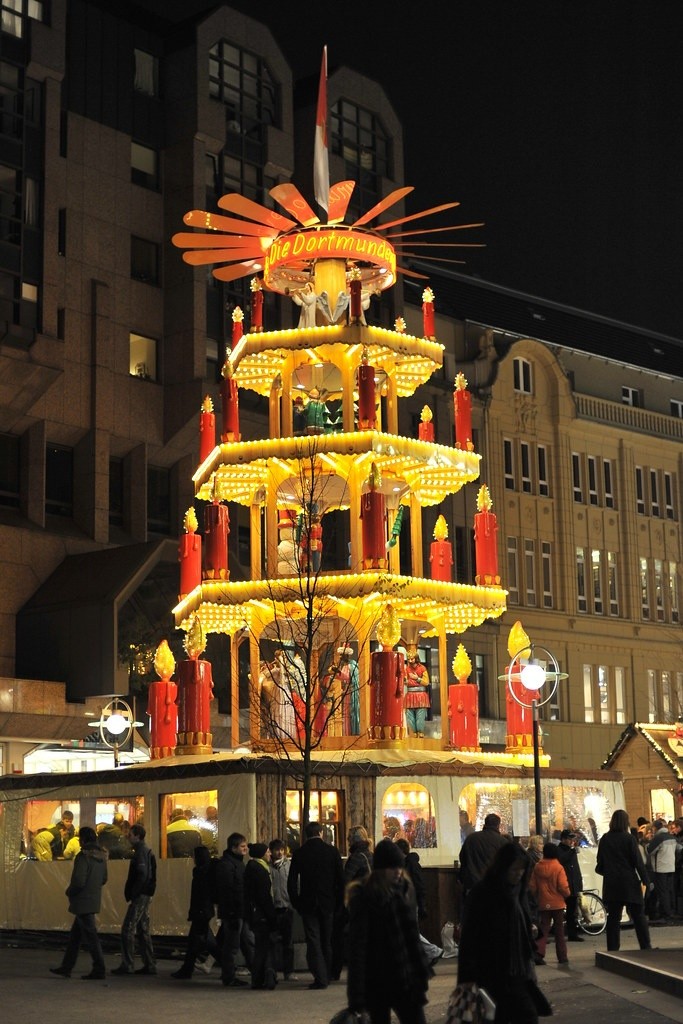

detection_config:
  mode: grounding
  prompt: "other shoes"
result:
[81,971,106,979]
[170,969,193,979]
[534,956,546,965]
[568,937,584,942]
[284,973,298,981]
[194,962,211,974]
[226,978,248,986]
[134,964,158,975]
[233,966,251,975]
[559,959,568,963]
[111,965,134,976]
[308,981,327,990]
[49,966,72,978]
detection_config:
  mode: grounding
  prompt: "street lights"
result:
[88,697,144,813]
[498,641,569,836]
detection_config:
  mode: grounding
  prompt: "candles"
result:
[359,349,376,420]
[419,405,435,443]
[220,359,239,433]
[232,306,244,351]
[358,461,387,559]
[250,276,263,327]
[506,620,539,736]
[200,395,216,463]
[178,506,202,595]
[454,372,472,443]
[446,643,478,748]
[428,514,454,582]
[175,616,215,733]
[145,640,179,748]
[473,484,499,576]
[202,478,230,571]
[394,316,407,334]
[422,287,435,336]
[368,604,405,726]
[351,266,362,316]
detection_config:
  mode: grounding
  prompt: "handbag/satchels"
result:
[329,1004,371,1024]
[595,857,605,876]
[446,981,496,1024]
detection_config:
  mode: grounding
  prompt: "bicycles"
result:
[531,888,609,942]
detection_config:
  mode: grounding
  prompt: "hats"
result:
[248,843,268,859]
[560,829,576,839]
[74,827,96,841]
[371,837,405,871]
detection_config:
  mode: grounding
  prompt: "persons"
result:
[331,647,361,735]
[458,810,476,843]
[111,825,158,975]
[290,283,316,329]
[597,810,654,951]
[343,838,437,1024]
[293,389,326,435]
[296,504,322,572]
[20,811,81,861]
[529,815,604,848]
[96,812,144,859]
[630,816,683,920]
[49,827,109,980]
[334,826,373,979]
[166,807,219,857]
[287,808,337,853]
[459,814,585,963]
[172,832,300,986]
[404,651,430,737]
[397,839,437,968]
[288,823,342,989]
[258,650,297,738]
[457,842,553,1024]
[383,816,437,848]
